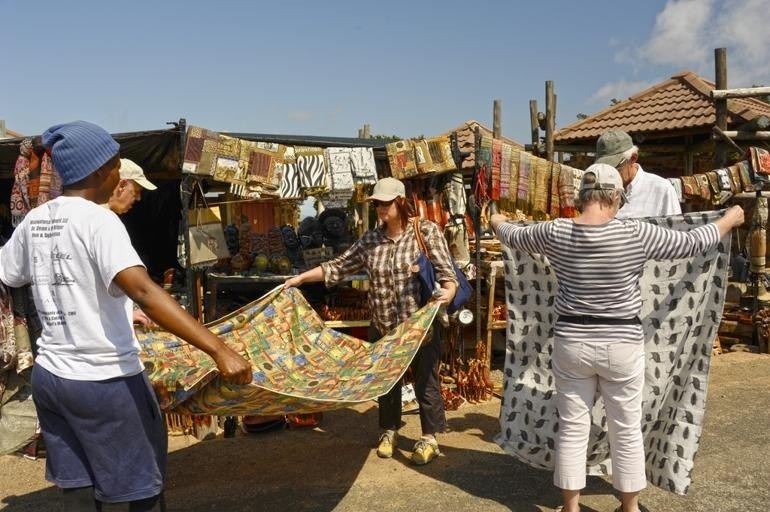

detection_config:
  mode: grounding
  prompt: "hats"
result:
[119,158,157,191]
[364,176,407,203]
[593,129,634,167]
[578,163,630,204]
[40,120,121,186]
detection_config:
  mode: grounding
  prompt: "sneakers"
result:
[411,436,440,465]
[377,428,400,458]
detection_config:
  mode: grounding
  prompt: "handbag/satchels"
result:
[177,181,218,271]
[188,181,232,263]
[412,216,473,314]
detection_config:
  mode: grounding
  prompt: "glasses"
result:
[373,199,393,208]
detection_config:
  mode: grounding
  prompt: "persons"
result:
[250,233,270,268]
[282,223,304,265]
[231,213,252,271]
[99,159,158,216]
[299,216,322,249]
[0,121,252,512]
[284,179,458,466]
[595,132,683,219]
[267,226,284,261]
[732,247,748,283]
[492,164,745,512]
[224,223,238,257]
[318,206,349,251]
